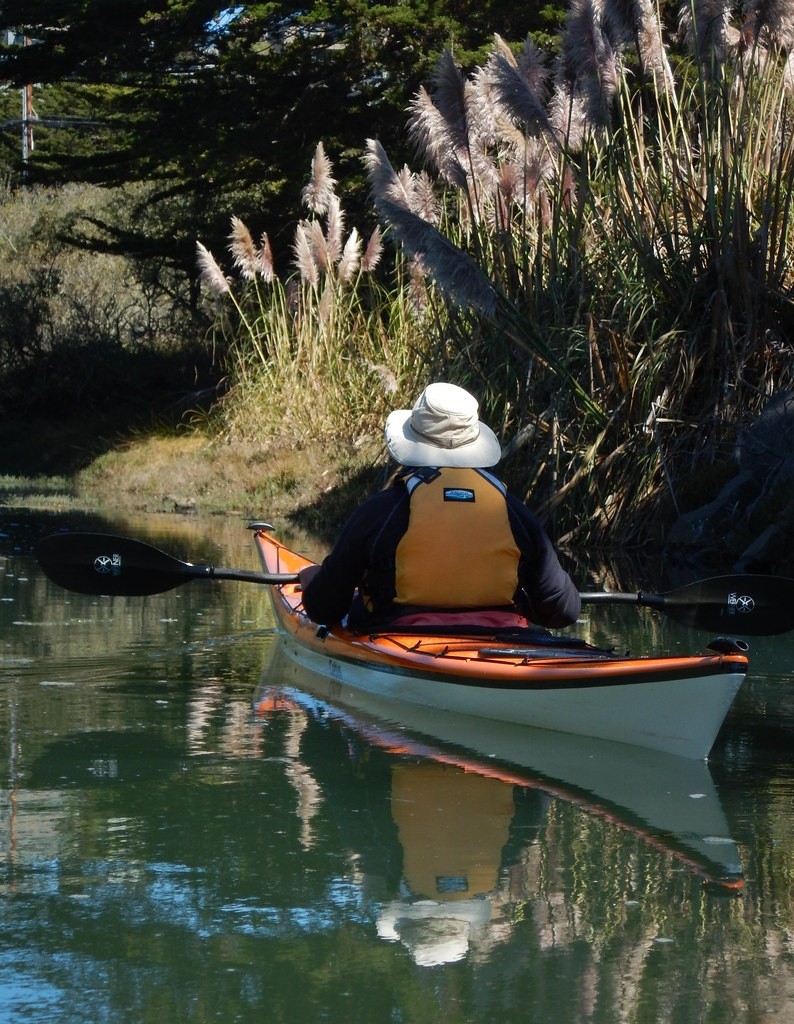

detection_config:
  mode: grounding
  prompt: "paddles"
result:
[30,531,794,640]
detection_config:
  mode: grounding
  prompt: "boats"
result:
[247,520,751,761]
[253,654,748,899]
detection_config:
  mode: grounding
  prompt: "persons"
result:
[299,381,582,629]
[300,721,545,967]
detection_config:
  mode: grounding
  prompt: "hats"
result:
[376,899,493,969]
[384,382,501,468]
